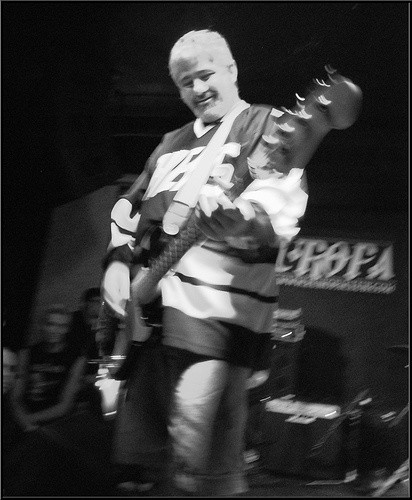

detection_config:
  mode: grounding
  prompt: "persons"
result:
[94,27,309,498]
[1,284,156,496]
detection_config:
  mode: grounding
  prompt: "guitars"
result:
[94,64,361,380]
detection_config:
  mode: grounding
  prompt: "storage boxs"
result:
[264,394,342,480]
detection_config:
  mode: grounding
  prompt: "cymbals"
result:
[387,344,409,354]
